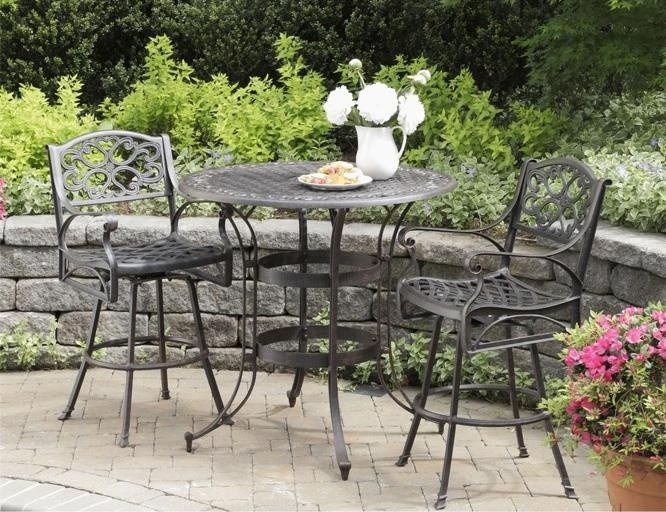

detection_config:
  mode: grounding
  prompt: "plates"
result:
[297,172,374,191]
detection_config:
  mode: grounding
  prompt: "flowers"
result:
[539,296,665,489]
[322,57,434,138]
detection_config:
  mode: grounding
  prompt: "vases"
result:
[598,445,665,511]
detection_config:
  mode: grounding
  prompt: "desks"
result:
[178,157,458,482]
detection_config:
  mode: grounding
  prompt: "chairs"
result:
[42,129,239,449]
[395,154,614,512]
